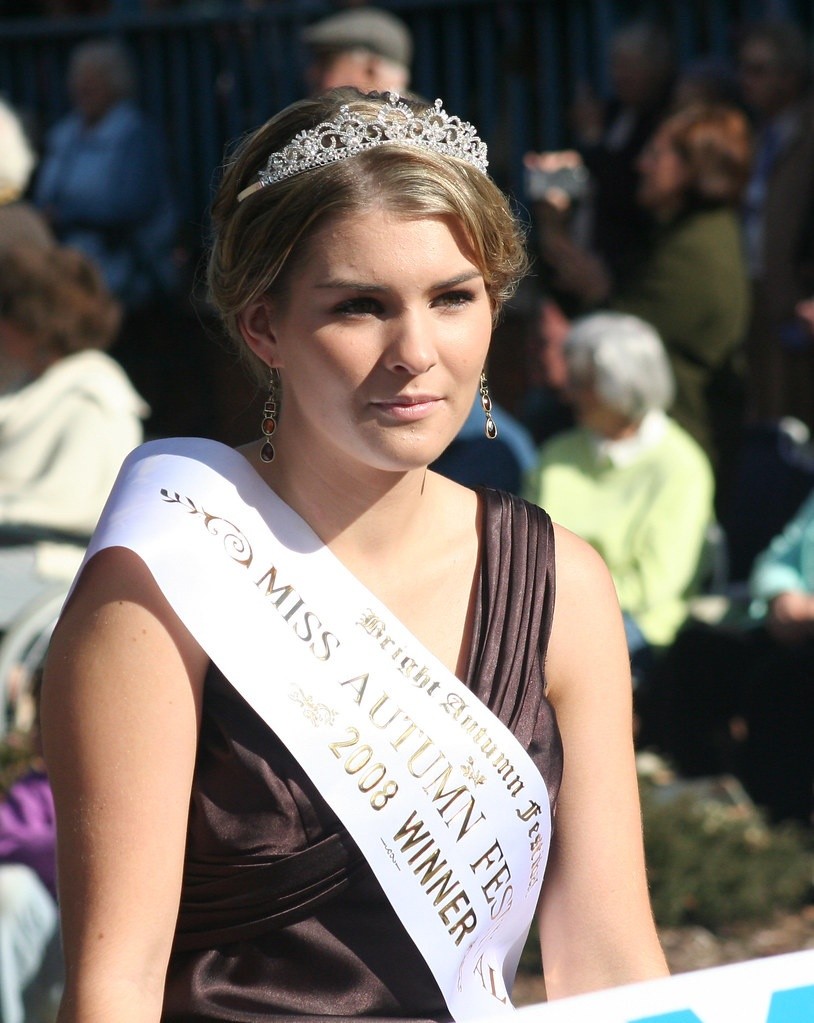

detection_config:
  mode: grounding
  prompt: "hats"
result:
[300,7,411,65]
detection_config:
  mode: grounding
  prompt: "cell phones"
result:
[525,164,588,198]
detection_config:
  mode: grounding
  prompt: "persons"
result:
[40,88,670,1023]
[299,10,814,817]
[0,56,188,1023]
